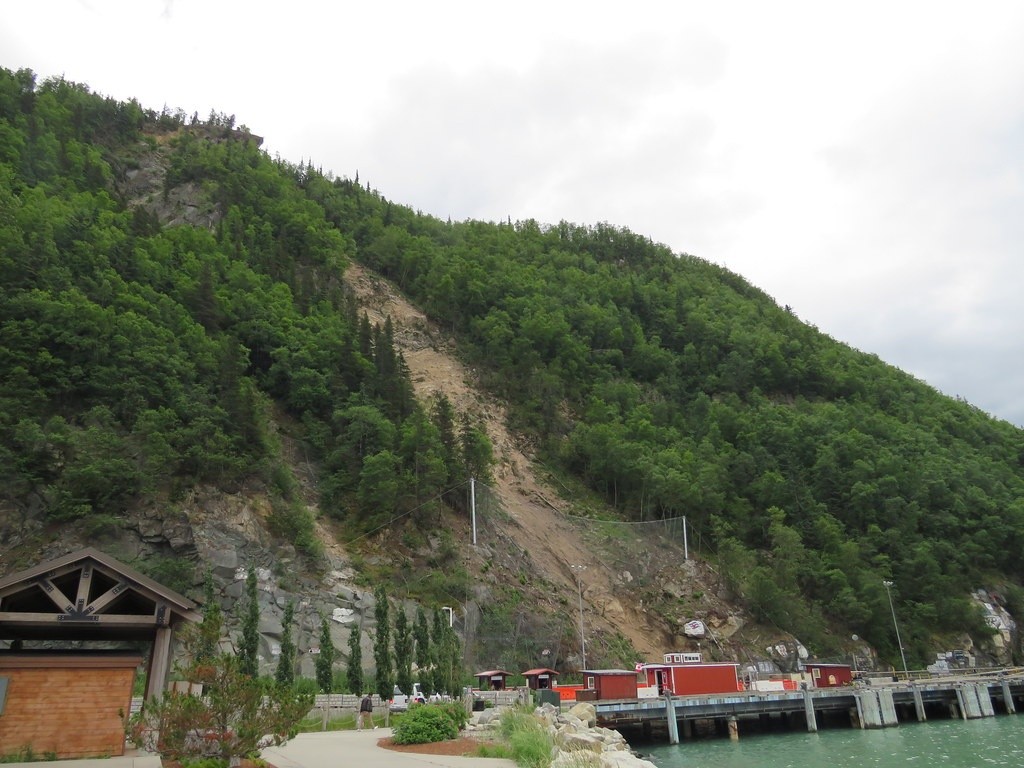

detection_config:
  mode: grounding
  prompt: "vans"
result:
[391,681,441,710]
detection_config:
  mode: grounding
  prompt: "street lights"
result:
[882,580,911,680]
[569,563,589,670]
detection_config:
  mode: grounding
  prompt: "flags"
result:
[635,663,643,672]
[688,622,700,629]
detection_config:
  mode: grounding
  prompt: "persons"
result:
[357,692,378,732]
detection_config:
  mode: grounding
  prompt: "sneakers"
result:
[373,726,378,730]
[356,729,363,732]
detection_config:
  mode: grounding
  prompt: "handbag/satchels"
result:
[356,715,365,728]
